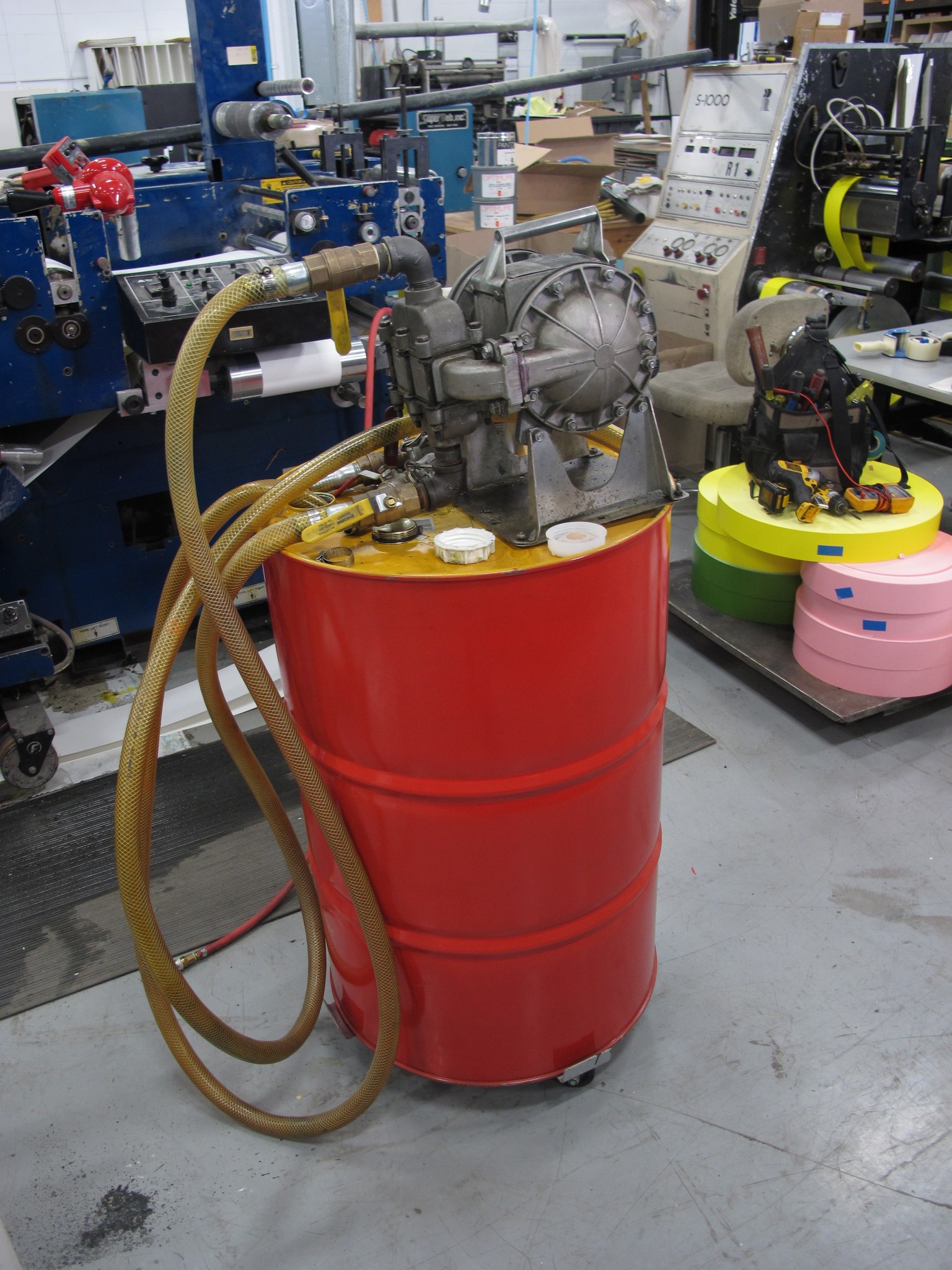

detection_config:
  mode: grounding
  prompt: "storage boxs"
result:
[512,142,619,215]
[791,9,851,59]
[514,117,617,166]
[565,106,620,116]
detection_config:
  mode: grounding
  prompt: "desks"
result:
[829,318,952,462]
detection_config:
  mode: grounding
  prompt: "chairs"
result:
[651,293,831,472]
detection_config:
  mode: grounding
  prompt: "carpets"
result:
[1,708,715,1021]
